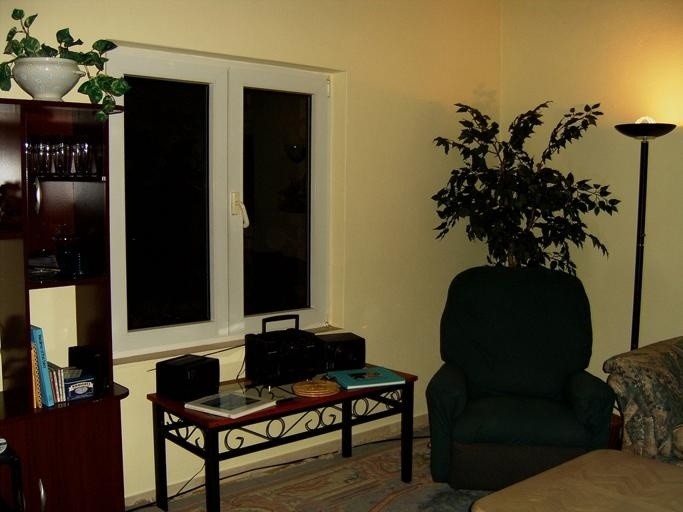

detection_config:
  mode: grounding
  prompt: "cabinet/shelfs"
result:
[0,98,130,511]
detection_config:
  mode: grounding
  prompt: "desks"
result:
[473,447,683,512]
[146,363,416,511]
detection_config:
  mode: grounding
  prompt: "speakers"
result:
[316,332,365,372]
[156,354,219,402]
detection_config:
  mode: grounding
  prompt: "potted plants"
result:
[0,6,127,124]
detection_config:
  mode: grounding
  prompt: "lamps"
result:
[611,112,678,350]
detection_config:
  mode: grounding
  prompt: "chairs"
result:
[425,264,617,491]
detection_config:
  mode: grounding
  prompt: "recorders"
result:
[245,314,316,388]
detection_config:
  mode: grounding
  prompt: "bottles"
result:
[31,142,94,176]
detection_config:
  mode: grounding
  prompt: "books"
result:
[29,322,94,410]
[331,365,405,391]
